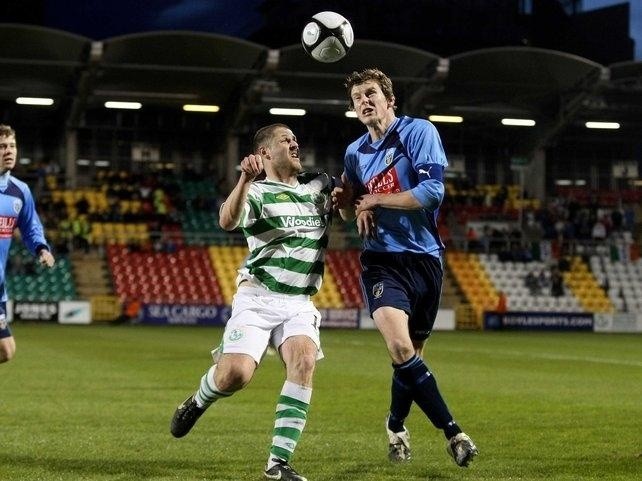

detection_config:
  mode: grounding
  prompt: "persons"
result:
[169,121,336,480]
[525,265,566,297]
[0,123,56,363]
[24,158,226,252]
[115,298,140,322]
[328,68,480,470]
[437,172,641,273]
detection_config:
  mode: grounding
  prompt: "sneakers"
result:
[447,432,479,468]
[385,416,411,461]
[264,462,307,481]
[170,390,205,438]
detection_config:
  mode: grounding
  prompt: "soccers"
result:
[302,9,354,65]
